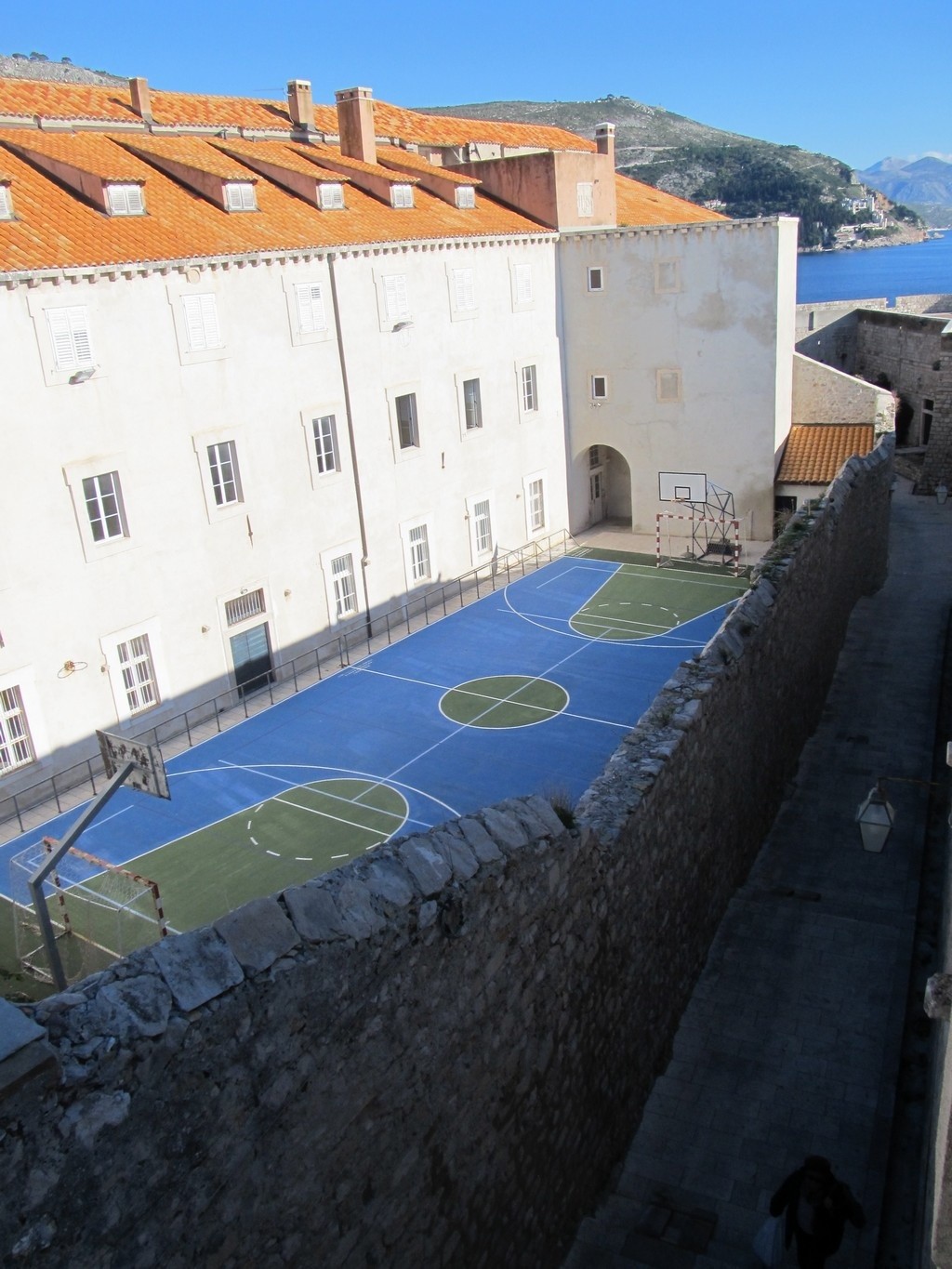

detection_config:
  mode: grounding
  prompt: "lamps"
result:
[854,778,952,852]
[935,479,952,504]
[395,322,414,331]
[72,369,95,384]
